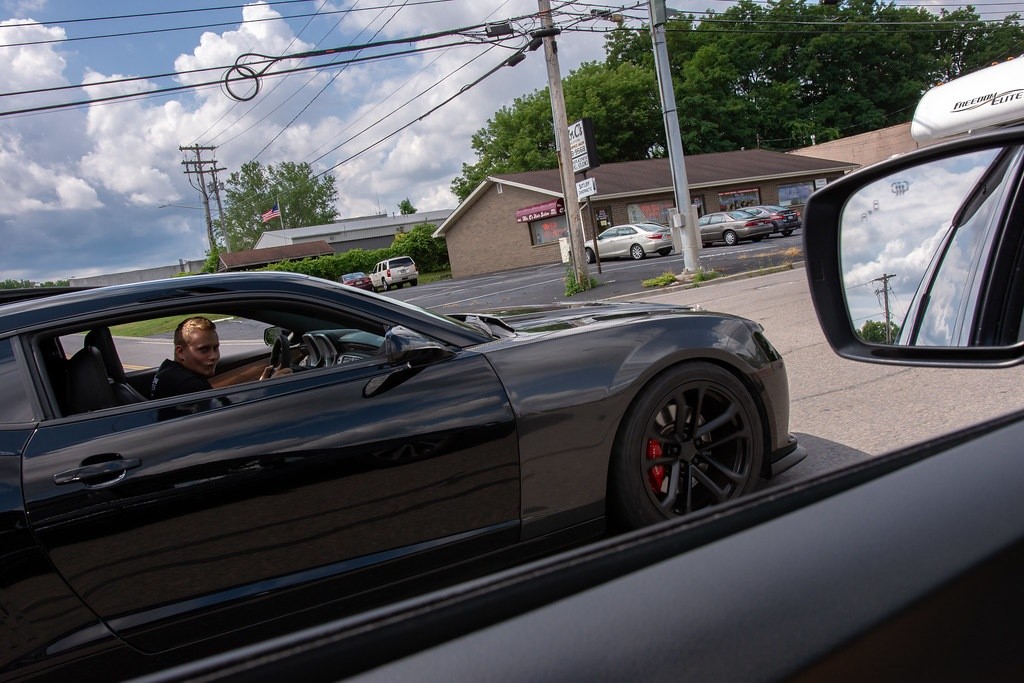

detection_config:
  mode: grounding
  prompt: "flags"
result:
[262,205,279,222]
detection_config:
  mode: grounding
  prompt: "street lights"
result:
[158,203,232,254]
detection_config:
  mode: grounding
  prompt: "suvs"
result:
[369,256,419,293]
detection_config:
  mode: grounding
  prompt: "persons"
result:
[149,317,293,399]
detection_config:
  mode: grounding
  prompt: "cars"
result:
[699,210,775,249]
[568,222,673,264]
[0,270,811,683]
[736,205,803,239]
[337,271,374,292]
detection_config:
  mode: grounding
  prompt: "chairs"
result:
[83,325,147,403]
[66,346,114,411]
